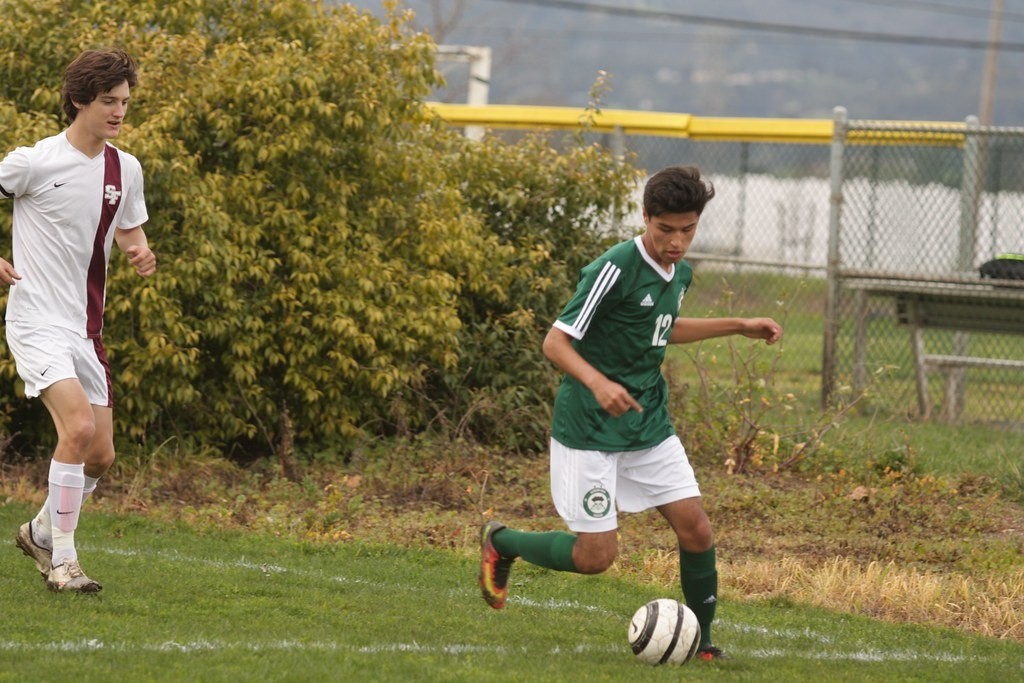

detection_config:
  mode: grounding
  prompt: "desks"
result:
[842,280,1024,404]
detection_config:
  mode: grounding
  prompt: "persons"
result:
[479,167,783,661]
[0,49,156,594]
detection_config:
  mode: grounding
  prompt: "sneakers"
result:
[698,644,731,662]
[46,560,102,593]
[15,520,52,578]
[480,520,516,609]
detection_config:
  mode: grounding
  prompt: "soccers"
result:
[627,597,703,667]
